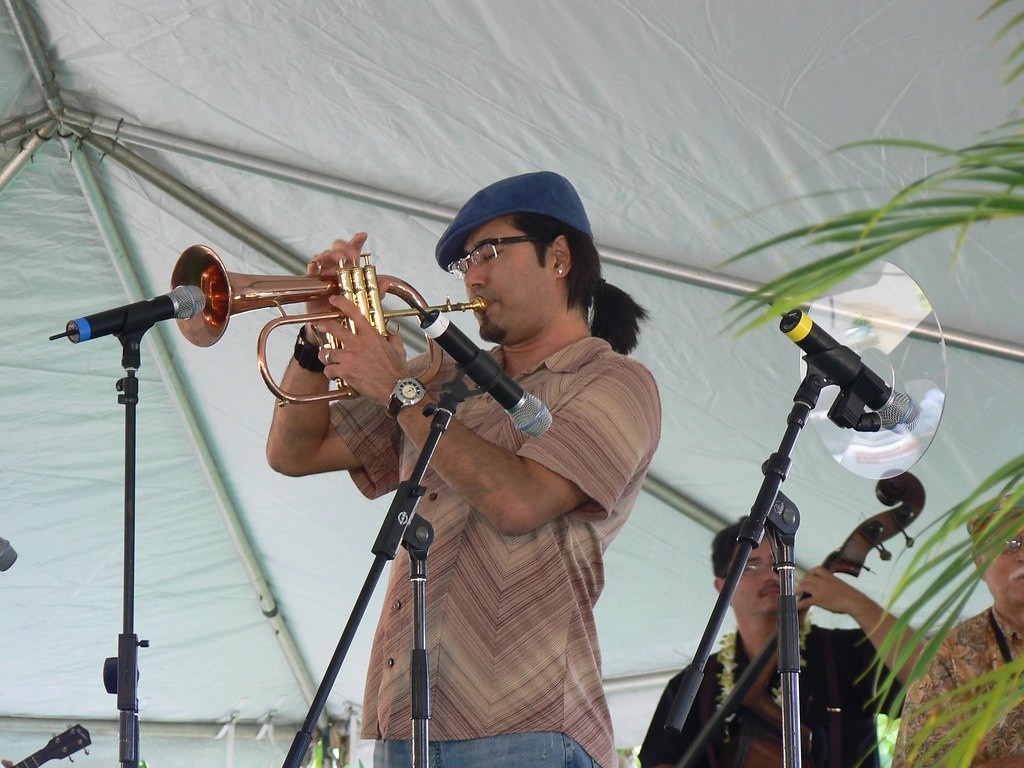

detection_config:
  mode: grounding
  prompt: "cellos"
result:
[660,467,926,768]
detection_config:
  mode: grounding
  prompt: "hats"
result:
[435,172,593,273]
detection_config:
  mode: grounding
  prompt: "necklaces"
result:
[716,610,811,742]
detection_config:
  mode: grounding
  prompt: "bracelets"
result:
[294,325,326,372]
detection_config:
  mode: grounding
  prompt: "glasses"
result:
[742,561,774,575]
[449,235,542,283]
[979,536,1024,555]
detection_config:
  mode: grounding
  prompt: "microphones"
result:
[409,299,553,437]
[778,309,922,435]
[49,285,206,344]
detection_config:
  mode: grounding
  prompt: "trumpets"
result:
[166,242,486,407]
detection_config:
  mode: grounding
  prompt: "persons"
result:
[891,492,1024,768]
[637,516,933,768]
[265,172,661,768]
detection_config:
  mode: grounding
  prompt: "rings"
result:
[325,350,332,364]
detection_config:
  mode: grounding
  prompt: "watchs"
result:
[384,377,427,420]
